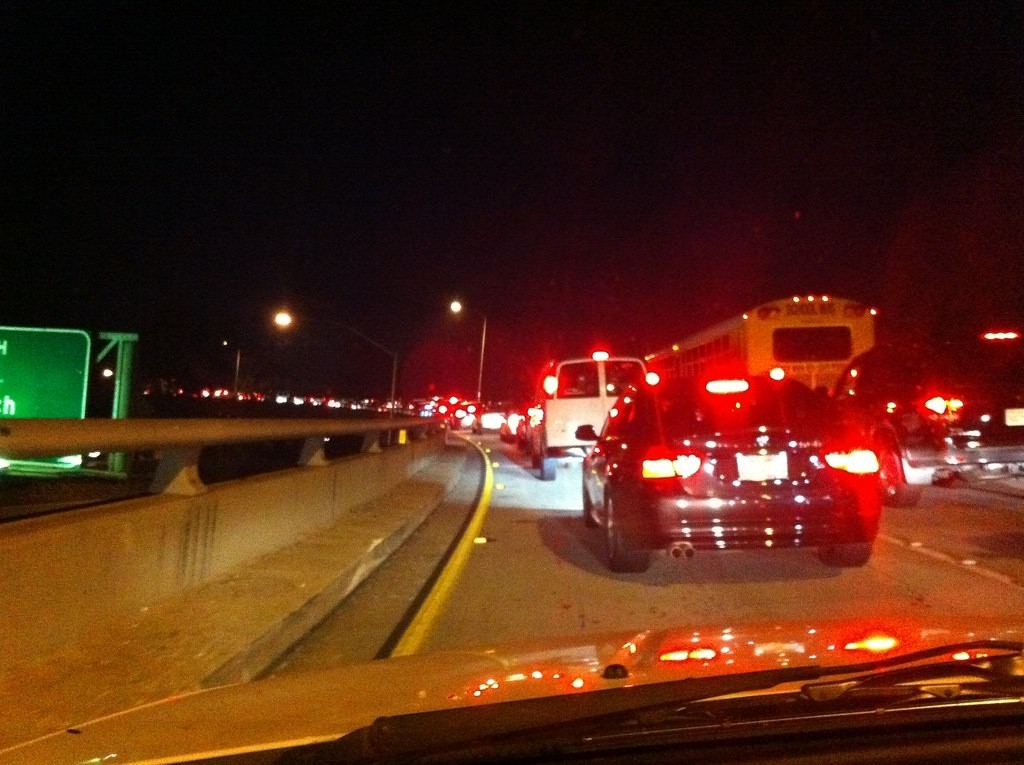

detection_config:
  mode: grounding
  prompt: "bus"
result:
[644,294,877,397]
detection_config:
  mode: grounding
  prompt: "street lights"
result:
[275,311,398,448]
[451,300,489,402]
[222,339,242,401]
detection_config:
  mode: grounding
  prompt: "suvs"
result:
[574,368,884,573]
[831,328,1024,508]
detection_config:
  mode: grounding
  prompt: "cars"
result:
[471,407,507,435]
[530,352,649,482]
[435,398,464,420]
[172,385,440,418]
[447,402,483,430]
[0,615,1024,765]
[500,405,529,446]
